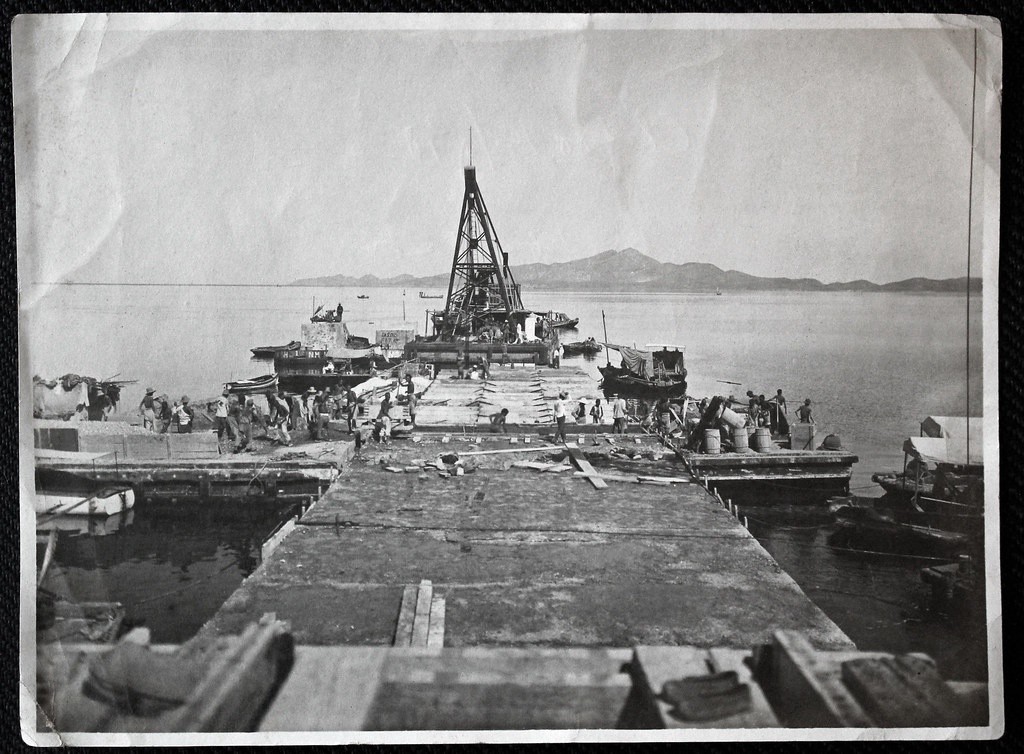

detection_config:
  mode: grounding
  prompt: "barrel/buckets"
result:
[733,428,749,453]
[755,428,772,452]
[705,428,721,454]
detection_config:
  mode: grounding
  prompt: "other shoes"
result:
[563,439,568,443]
[552,438,559,445]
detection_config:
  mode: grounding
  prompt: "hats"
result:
[803,399,811,405]
[307,387,316,393]
[504,319,508,322]
[181,395,190,402]
[145,387,156,394]
[578,397,587,405]
[559,391,566,398]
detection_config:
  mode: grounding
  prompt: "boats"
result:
[37,588,125,645]
[357,295,369,298]
[34,465,137,515]
[597,341,688,399]
[534,312,578,329]
[683,395,858,505]
[419,292,443,298]
[561,341,602,354]
[871,415,985,518]
[825,495,985,555]
[35,511,135,544]
[222,372,279,393]
[252,341,302,356]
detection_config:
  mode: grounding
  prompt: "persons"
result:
[695,399,710,418]
[489,408,509,433]
[746,388,787,433]
[655,394,675,439]
[457,350,492,380]
[92,387,114,422]
[138,374,418,455]
[590,398,604,424]
[795,398,817,426]
[500,319,524,345]
[571,397,587,424]
[612,393,628,434]
[553,392,568,445]
[547,343,564,369]
